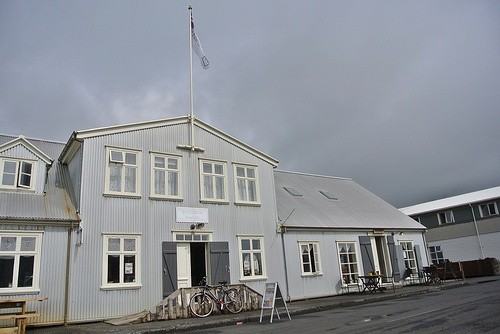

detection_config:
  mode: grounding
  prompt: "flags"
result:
[189,8,211,72]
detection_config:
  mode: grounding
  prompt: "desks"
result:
[0,297,49,334]
[418,266,438,286]
[357,275,384,294]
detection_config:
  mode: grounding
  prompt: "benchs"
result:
[0,314,40,334]
[0,311,36,327]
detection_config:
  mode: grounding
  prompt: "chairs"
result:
[379,277,396,295]
[346,280,361,295]
[405,266,423,285]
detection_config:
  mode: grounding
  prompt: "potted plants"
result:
[374,271,379,276]
[368,271,373,276]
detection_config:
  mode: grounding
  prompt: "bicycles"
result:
[190,276,243,317]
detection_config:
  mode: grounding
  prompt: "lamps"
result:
[196,222,205,229]
